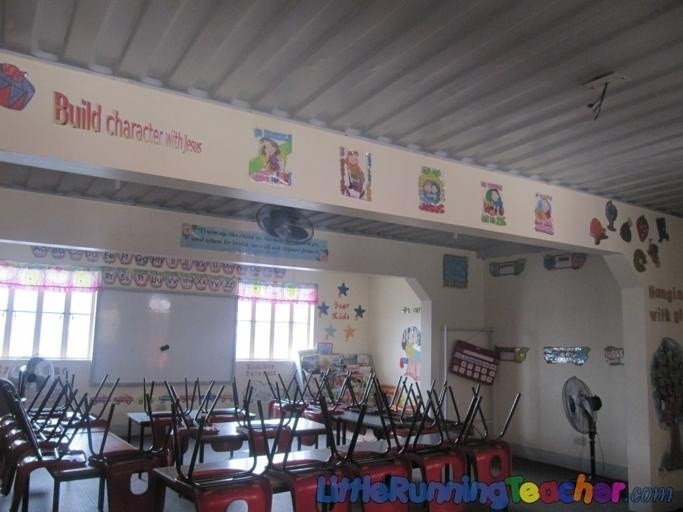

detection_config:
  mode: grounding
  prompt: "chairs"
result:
[1,369,523,512]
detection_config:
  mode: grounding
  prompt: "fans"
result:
[255,206,315,244]
[562,376,605,496]
[6,353,55,400]
